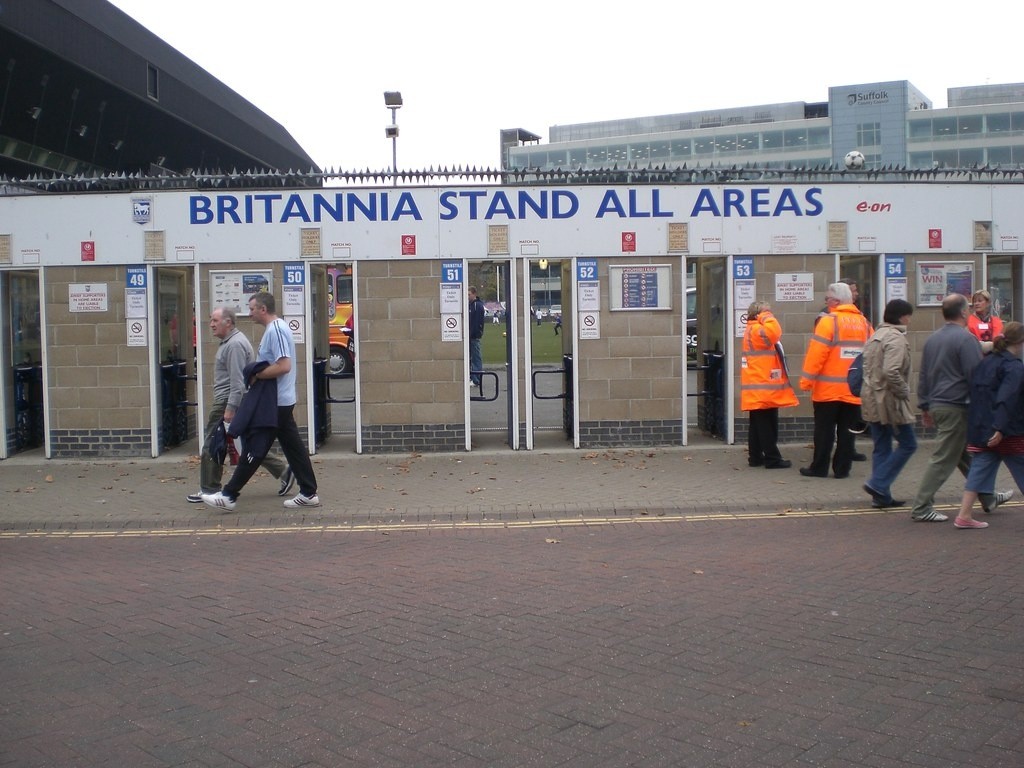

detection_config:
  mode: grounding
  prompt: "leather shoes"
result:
[835,473,849,479]
[871,499,906,508]
[749,457,765,466]
[765,460,792,468]
[800,467,828,476]
[851,451,866,462]
[863,483,893,506]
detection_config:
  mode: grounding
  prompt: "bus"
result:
[319,266,354,376]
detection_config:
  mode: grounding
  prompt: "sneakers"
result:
[186,492,208,503]
[470,381,478,388]
[278,465,294,497]
[953,515,988,529]
[284,493,320,508]
[983,488,1014,514]
[201,492,236,512]
[911,511,948,522]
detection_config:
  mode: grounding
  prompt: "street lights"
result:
[383,90,403,173]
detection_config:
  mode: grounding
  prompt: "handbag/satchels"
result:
[848,353,865,399]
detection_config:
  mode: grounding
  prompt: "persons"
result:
[493,310,500,325]
[861,298,918,508]
[553,312,562,335]
[798,279,875,479]
[740,301,799,469]
[186,292,320,513]
[468,287,484,387]
[536,308,542,325]
[910,290,1024,529]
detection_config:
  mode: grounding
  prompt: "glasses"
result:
[825,296,834,300]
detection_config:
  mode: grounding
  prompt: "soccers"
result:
[844,151,866,169]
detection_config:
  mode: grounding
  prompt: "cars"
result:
[531,306,547,317]
[168,304,197,359]
[549,305,562,317]
[686,287,697,360]
